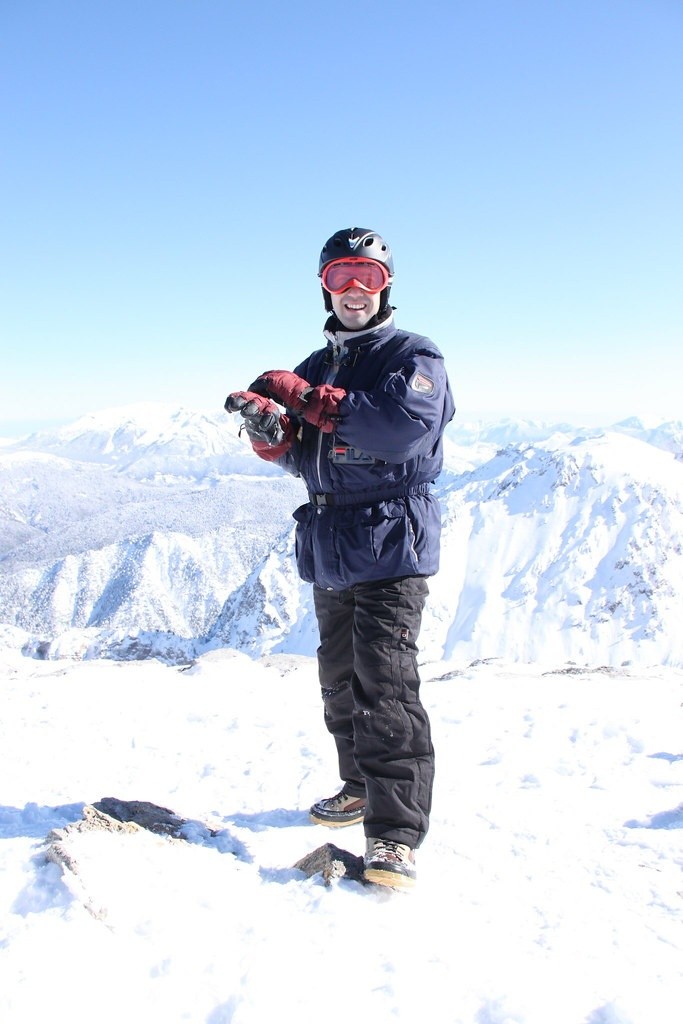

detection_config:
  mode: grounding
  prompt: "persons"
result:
[225,227,456,884]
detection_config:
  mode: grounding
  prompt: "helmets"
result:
[317,227,395,312]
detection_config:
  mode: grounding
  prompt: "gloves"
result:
[224,369,344,461]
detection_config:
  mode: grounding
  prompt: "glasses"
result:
[322,256,390,294]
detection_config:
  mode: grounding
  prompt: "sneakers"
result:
[363,835,417,886]
[309,790,367,827]
